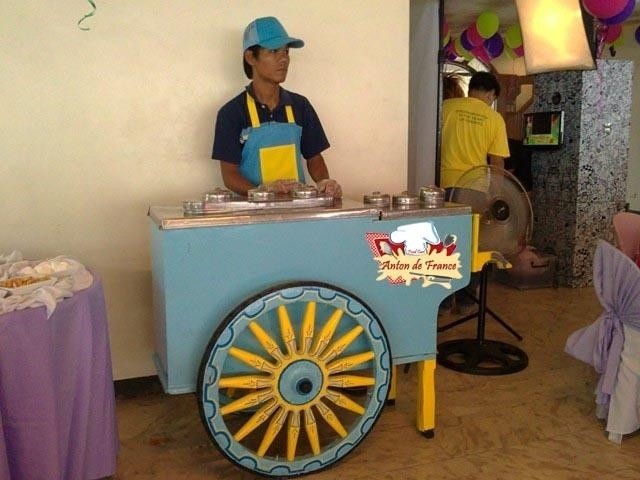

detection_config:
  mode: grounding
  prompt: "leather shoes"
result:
[454,287,479,305]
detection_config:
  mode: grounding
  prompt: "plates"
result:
[0,274,58,294]
[0,289,8,298]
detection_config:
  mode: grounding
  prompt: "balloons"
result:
[582,0,628,19]
[442,11,524,64]
[599,0,635,25]
[602,24,622,44]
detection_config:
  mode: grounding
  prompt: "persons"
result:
[211,16,342,206]
[441,70,511,304]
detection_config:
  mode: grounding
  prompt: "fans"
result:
[436,165,532,374]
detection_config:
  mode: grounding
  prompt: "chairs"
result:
[613,214,639,259]
[592,239,640,444]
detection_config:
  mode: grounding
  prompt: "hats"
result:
[240,15,305,50]
